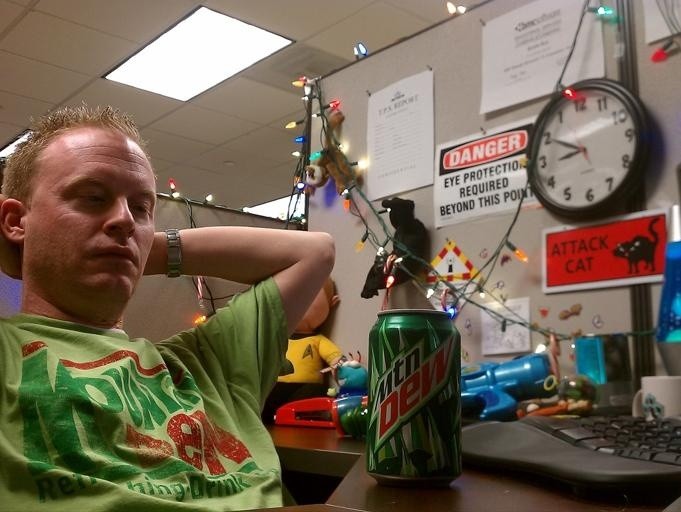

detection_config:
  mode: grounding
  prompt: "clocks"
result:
[531,78,654,220]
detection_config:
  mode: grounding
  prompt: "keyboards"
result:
[459,415,681,507]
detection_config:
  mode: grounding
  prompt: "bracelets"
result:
[166,226,182,277]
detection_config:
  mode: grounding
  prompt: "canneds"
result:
[364,309,463,488]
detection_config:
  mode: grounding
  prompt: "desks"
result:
[265,421,681,512]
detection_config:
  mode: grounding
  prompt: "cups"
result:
[631,375,681,421]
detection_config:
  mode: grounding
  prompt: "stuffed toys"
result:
[380,198,429,288]
[333,348,369,419]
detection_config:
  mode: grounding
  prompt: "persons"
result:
[1,101,337,512]
[259,278,342,424]
